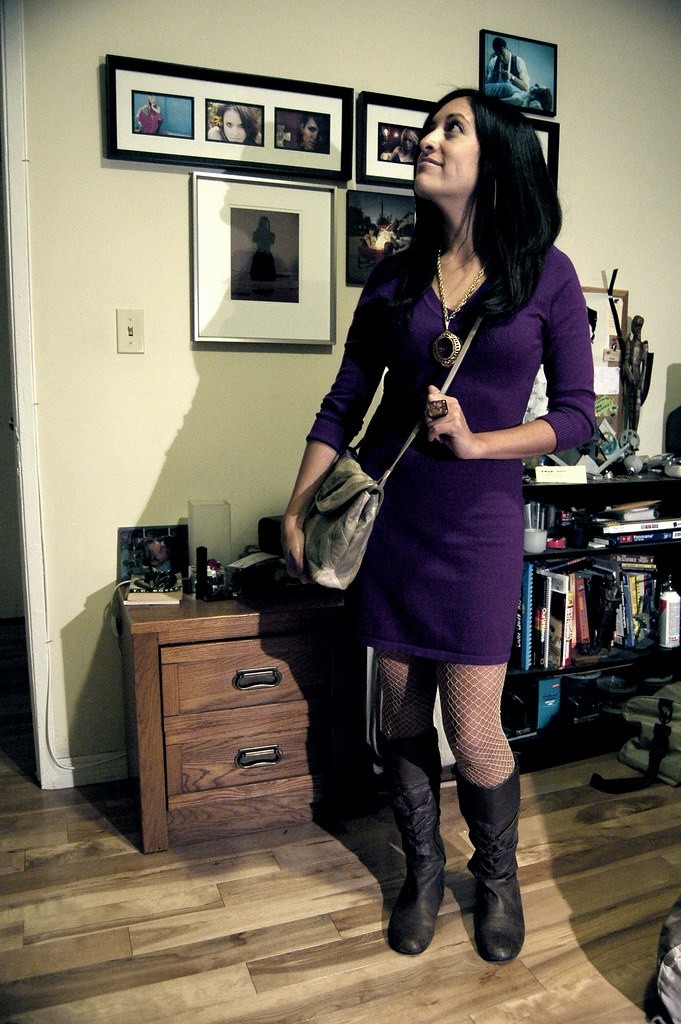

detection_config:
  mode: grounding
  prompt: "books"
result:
[513,555,658,670]
[123,572,183,606]
[593,508,681,544]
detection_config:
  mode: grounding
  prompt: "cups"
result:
[522,501,548,554]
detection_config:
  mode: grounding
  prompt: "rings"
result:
[425,400,448,419]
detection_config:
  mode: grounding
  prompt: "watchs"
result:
[512,75,515,83]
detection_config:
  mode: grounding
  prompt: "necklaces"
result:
[433,249,487,367]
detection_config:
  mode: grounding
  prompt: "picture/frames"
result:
[345,189,416,287]
[525,118,560,192]
[523,285,628,440]
[356,92,439,188]
[192,170,338,346]
[105,53,353,183]
[479,28,558,117]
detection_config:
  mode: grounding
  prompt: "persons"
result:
[380,129,418,162]
[624,315,649,452]
[298,113,326,152]
[122,536,175,580]
[485,37,552,110]
[136,95,163,134]
[208,105,261,144]
[281,88,595,963]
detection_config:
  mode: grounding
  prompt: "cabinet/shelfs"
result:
[439,472,681,774]
[115,586,382,855]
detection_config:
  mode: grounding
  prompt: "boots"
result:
[379,735,448,955]
[452,753,526,966]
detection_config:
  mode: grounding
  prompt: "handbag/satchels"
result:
[304,447,388,590]
